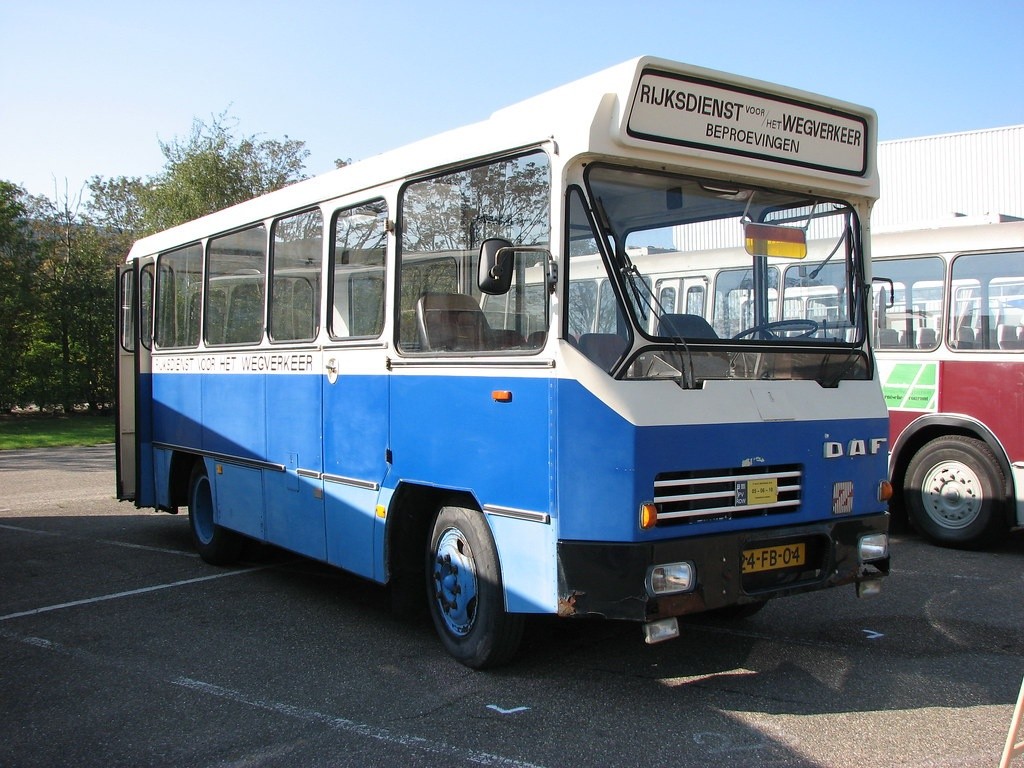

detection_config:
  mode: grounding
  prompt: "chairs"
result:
[185,281,1024,396]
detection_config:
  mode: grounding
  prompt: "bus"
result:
[476,212,1024,543]
[113,57,895,672]
